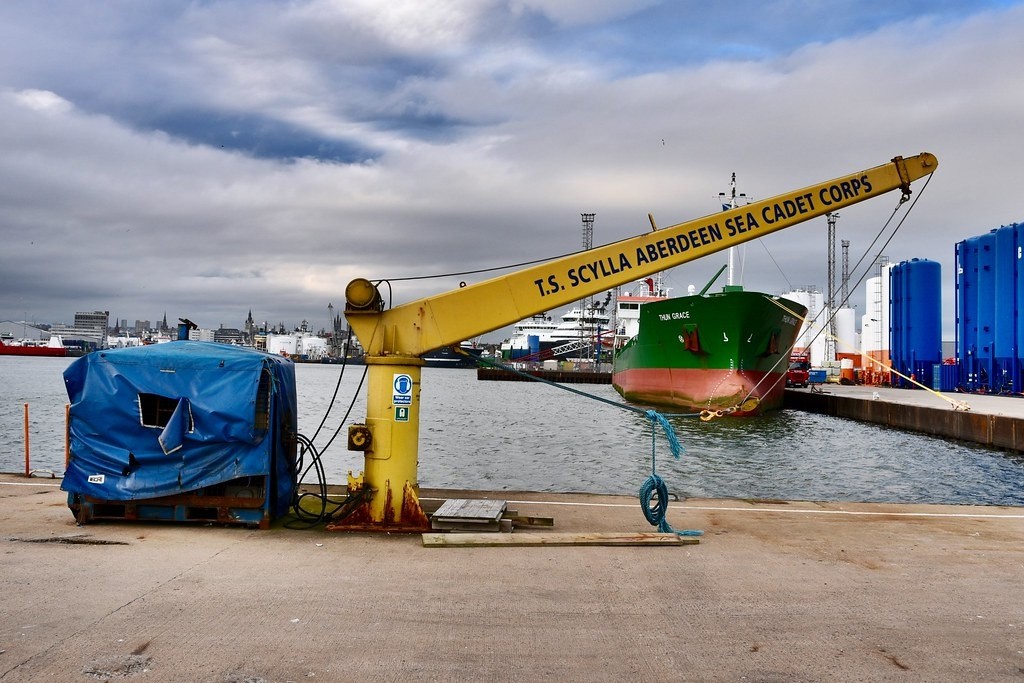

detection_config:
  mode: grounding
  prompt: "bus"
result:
[22,341,48,348]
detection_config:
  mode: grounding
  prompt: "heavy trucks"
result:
[786,347,811,388]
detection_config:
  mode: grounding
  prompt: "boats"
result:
[499,312,560,351]
[612,172,809,417]
[0,335,82,357]
[511,304,628,364]
